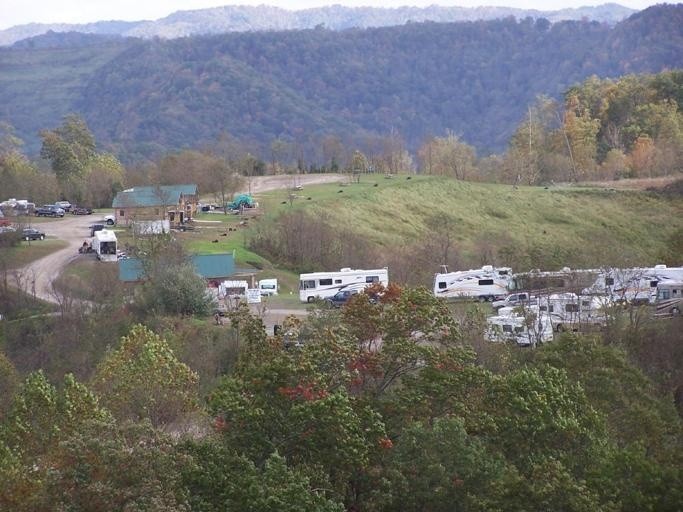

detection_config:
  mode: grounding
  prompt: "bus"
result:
[654,279,682,316]
[487,290,616,348]
[582,264,682,302]
[432,264,512,302]
[93,229,118,262]
[298,266,389,303]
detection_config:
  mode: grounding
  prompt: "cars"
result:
[16,227,45,241]
[0,198,93,218]
